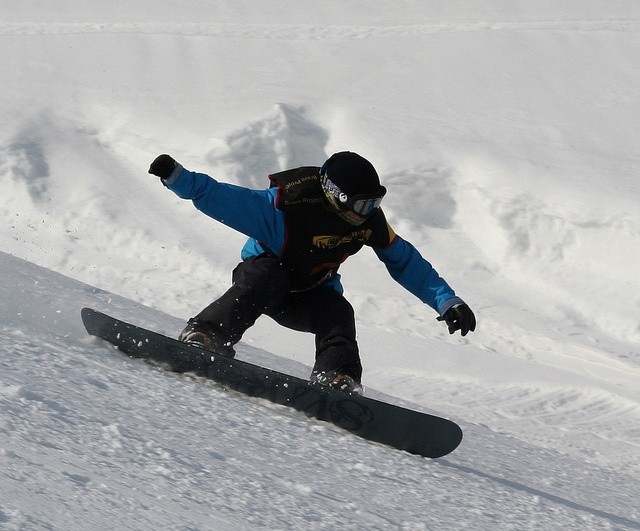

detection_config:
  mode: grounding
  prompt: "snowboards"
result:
[82,306,471,464]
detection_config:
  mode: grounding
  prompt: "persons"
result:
[149,152,476,397]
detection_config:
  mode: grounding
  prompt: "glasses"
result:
[347,187,387,219]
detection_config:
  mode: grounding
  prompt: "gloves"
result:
[443,302,477,336]
[148,153,176,179]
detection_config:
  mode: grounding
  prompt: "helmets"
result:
[320,150,381,213]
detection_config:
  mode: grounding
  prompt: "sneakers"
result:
[178,324,236,358]
[311,368,363,395]
